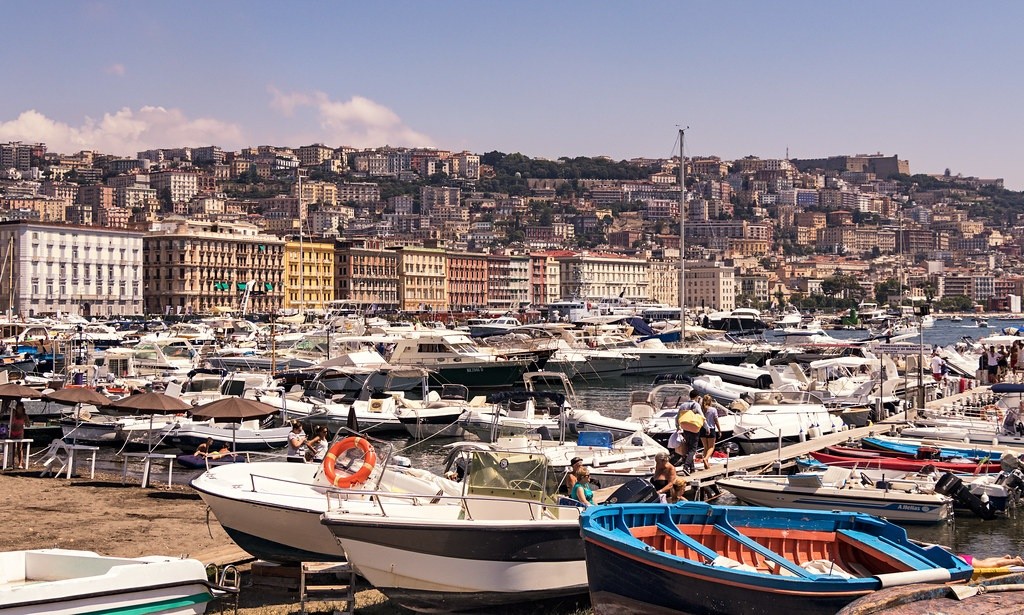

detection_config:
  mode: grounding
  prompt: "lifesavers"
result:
[323,436,377,489]
[494,354,509,362]
[587,302,593,309]
[981,403,1004,423]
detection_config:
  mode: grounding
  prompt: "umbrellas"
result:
[0,382,281,486]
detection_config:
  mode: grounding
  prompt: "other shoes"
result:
[682,465,690,476]
[703,458,709,469]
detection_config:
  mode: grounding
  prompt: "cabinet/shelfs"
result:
[300,562,355,615]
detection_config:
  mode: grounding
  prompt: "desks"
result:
[48,444,99,480]
[0,439,33,471]
[122,453,176,489]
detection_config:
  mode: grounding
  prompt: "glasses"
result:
[322,430,328,434]
[582,474,591,479]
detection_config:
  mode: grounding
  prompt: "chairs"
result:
[33,439,71,478]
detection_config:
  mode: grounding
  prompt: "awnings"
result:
[215,282,229,289]
[265,284,272,289]
[237,284,246,289]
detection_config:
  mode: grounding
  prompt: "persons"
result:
[979,352,988,370]
[305,425,328,462]
[196,437,213,460]
[700,395,722,469]
[0,398,12,438]
[1010,341,1018,373]
[667,479,687,503]
[286,422,307,463]
[7,402,30,470]
[987,346,1004,383]
[932,352,943,380]
[941,358,948,386]
[571,468,594,508]
[219,443,231,455]
[1018,344,1024,364]
[998,346,1008,372]
[956,553,1024,568]
[566,457,583,496]
[676,390,710,476]
[653,452,678,494]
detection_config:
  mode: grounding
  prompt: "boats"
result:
[0,124,1024,615]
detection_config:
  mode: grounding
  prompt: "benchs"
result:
[713,523,814,578]
[658,523,719,561]
[837,529,935,572]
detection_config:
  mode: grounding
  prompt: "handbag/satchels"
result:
[667,429,686,455]
[678,403,705,433]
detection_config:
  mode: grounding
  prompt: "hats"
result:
[570,457,583,467]
